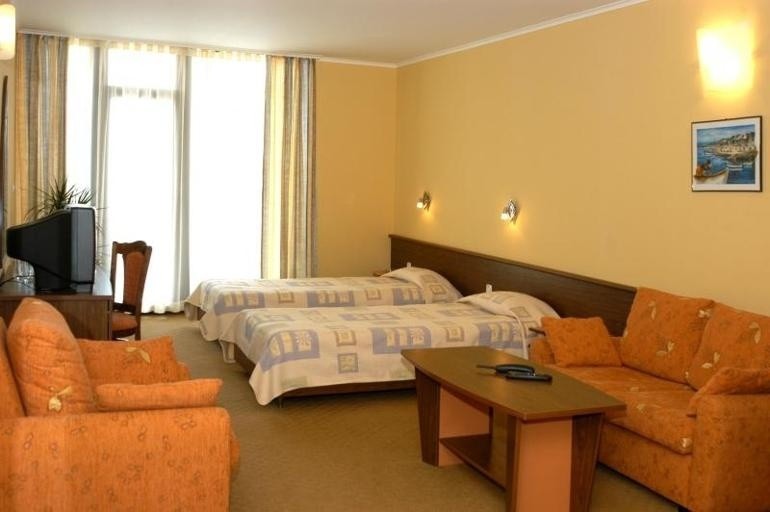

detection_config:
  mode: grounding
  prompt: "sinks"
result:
[528,280,770,512]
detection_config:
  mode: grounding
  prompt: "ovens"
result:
[181,264,463,343]
[220,285,566,406]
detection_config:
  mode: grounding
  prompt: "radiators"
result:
[507,371,553,381]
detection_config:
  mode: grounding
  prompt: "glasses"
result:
[416,190,430,211]
[502,199,516,223]
[1,0,17,59]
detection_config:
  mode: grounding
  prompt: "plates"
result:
[1,267,115,342]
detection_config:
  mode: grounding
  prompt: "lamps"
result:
[0,294,241,512]
[105,239,153,341]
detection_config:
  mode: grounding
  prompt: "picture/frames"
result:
[403,345,625,512]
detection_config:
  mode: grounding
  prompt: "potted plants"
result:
[690,114,764,192]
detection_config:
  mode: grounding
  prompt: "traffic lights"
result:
[686,364,770,421]
[95,373,222,410]
[541,314,622,368]
[382,266,445,285]
[458,288,557,318]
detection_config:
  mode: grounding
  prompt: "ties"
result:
[6,206,95,294]
